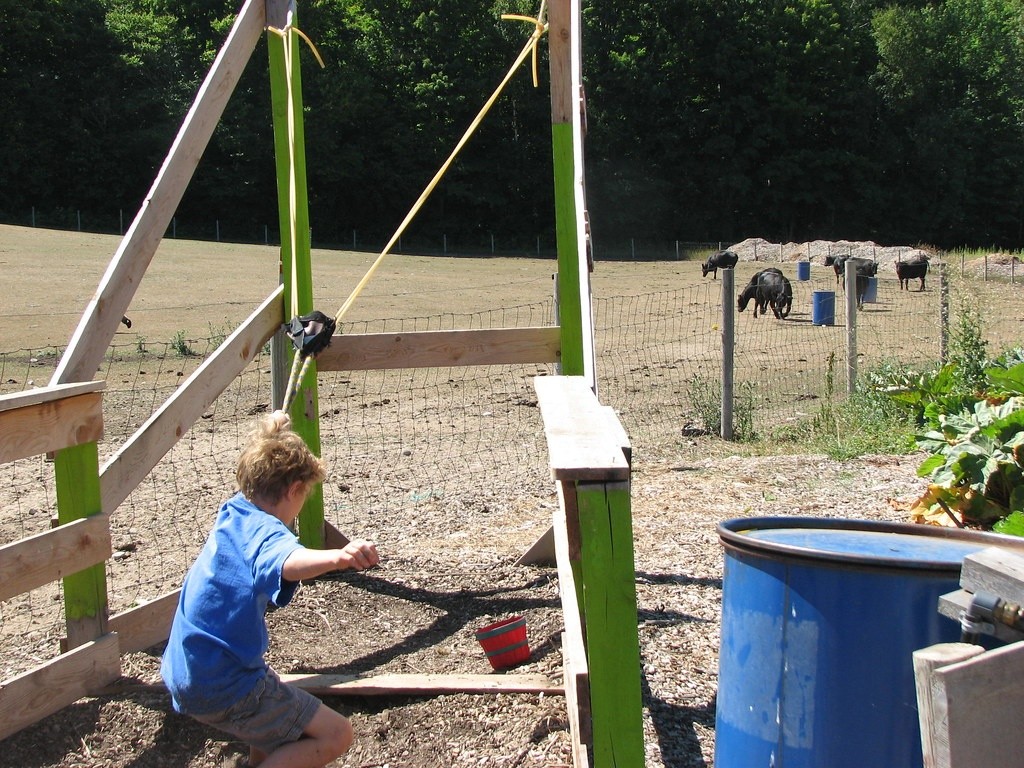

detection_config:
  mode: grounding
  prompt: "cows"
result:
[824,255,931,311]
[702,250,738,279]
[737,268,793,320]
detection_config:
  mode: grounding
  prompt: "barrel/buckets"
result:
[861,276,877,303]
[798,260,810,281]
[715,517,1024,768]
[475,615,532,671]
[812,290,836,325]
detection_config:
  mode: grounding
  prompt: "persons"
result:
[159,414,380,768]
